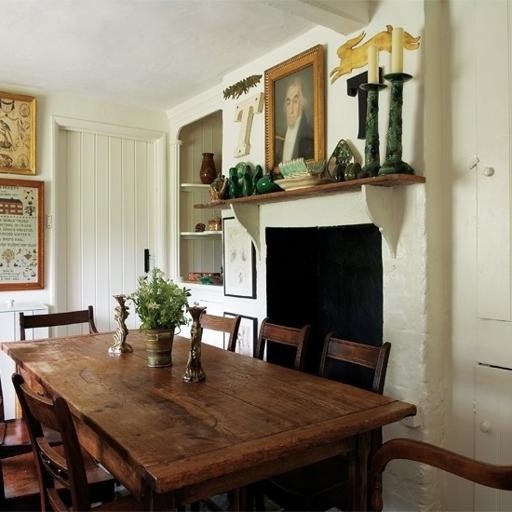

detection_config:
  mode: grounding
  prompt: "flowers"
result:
[127,263,193,332]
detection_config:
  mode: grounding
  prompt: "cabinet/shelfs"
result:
[177,177,223,286]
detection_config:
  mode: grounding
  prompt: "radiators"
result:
[1,300,49,422]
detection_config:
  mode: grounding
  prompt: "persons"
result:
[278,76,314,163]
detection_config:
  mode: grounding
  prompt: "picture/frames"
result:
[220,215,256,301]
[0,91,39,177]
[0,176,47,293]
[221,311,259,356]
[260,43,328,180]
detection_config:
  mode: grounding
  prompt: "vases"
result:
[138,328,176,370]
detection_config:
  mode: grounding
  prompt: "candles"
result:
[366,41,382,85]
[389,23,407,73]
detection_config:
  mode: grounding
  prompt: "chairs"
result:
[249,315,314,373]
[239,329,395,511]
[17,304,102,344]
[0,376,64,459]
[196,311,244,355]
[0,442,117,511]
[9,371,151,512]
